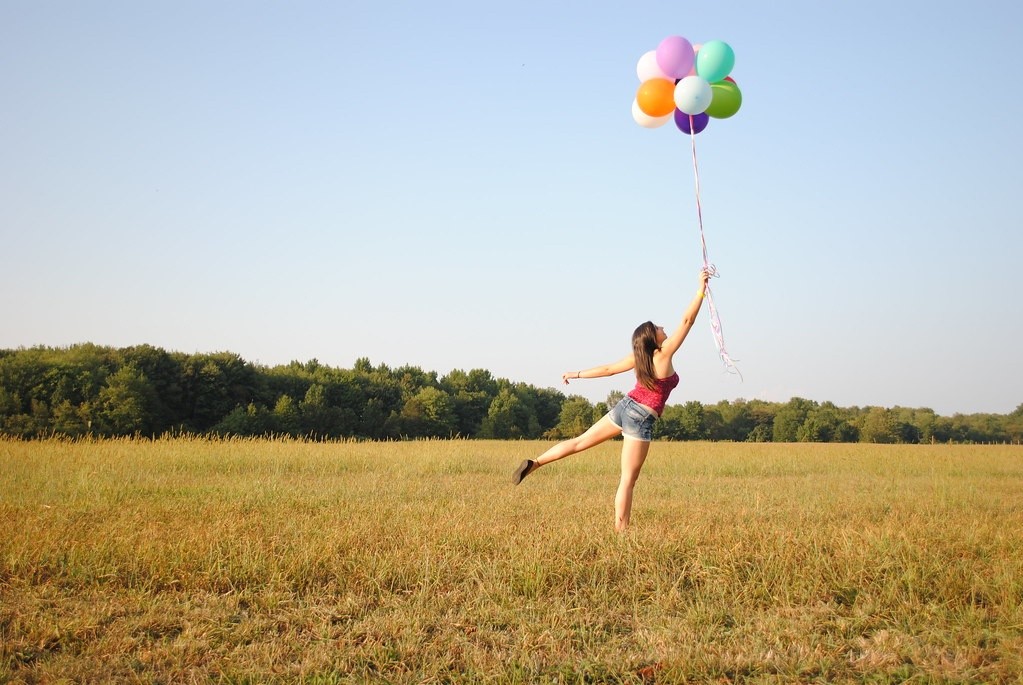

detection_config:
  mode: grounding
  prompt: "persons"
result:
[513,264,710,532]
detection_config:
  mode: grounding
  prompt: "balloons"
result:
[632,34,742,133]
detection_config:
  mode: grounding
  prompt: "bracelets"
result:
[578,371,580,378]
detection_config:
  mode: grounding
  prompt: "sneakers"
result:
[512,459,533,486]
